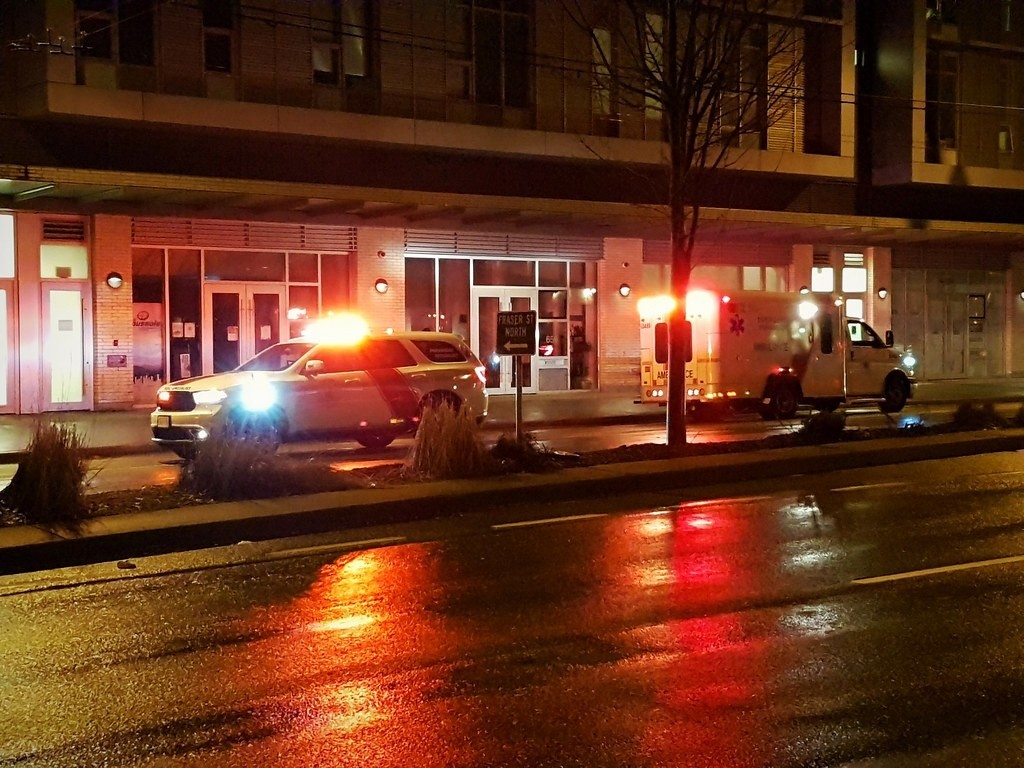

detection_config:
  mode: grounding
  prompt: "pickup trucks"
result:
[151,329,488,462]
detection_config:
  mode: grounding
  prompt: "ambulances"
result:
[633,289,917,421]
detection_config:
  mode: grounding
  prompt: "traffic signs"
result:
[496,311,536,355]
